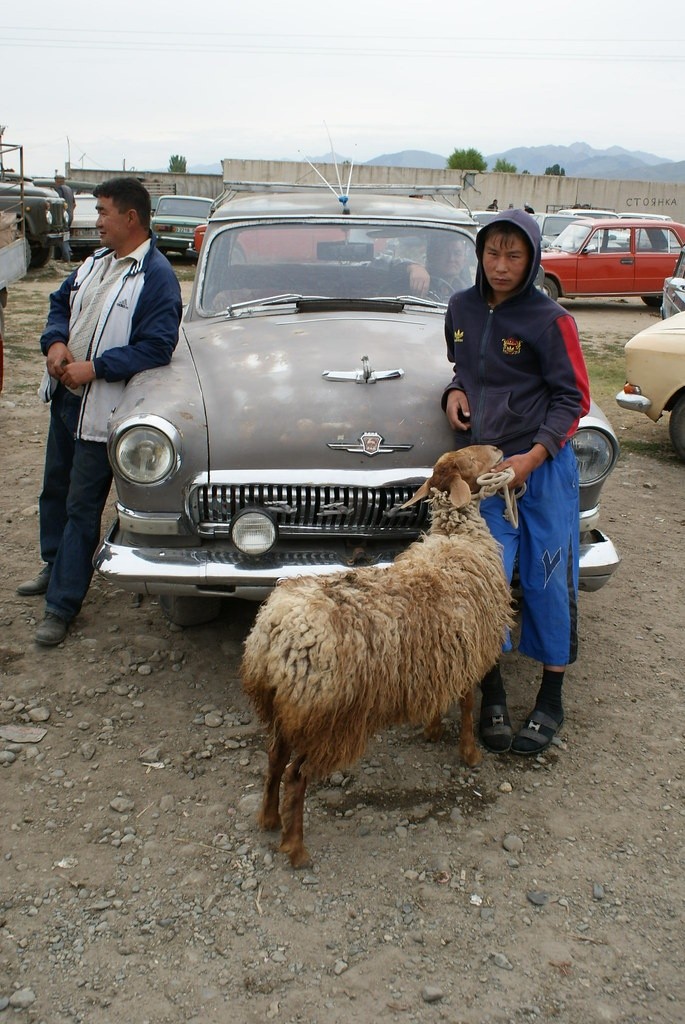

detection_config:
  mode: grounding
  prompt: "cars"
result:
[0,140,216,395]
[531,218,685,302]
[658,242,685,321]
[88,190,624,628]
[615,309,685,467]
[458,207,682,254]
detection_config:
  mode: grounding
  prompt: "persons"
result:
[54,176,76,262]
[15,177,182,645]
[508,203,514,209]
[524,203,535,214]
[486,199,498,212]
[368,230,467,303]
[439,208,590,756]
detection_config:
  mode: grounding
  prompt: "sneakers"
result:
[15,566,51,595]
[34,611,76,645]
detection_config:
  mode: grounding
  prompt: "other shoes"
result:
[57,259,70,263]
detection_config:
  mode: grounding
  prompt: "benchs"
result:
[215,265,389,293]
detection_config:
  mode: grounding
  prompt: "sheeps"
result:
[242,445,505,872]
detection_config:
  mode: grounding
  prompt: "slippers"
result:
[479,696,513,754]
[510,708,564,755]
